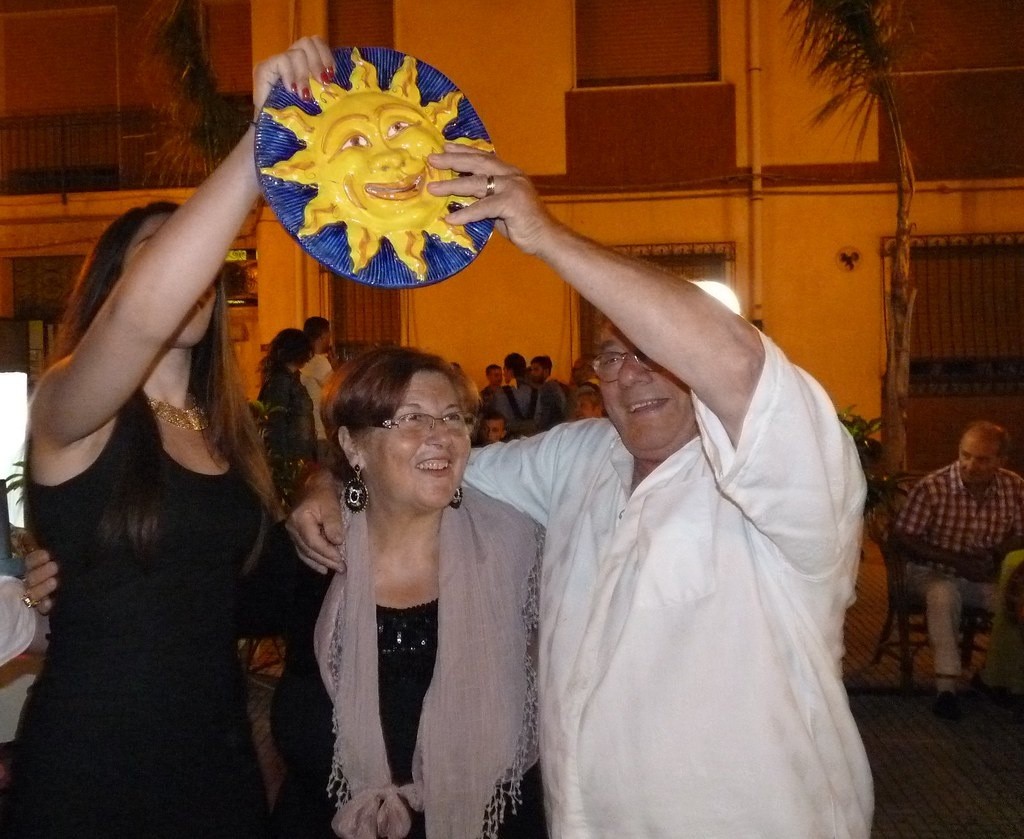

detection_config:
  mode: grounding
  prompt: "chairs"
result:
[866,474,996,699]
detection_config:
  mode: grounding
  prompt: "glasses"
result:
[590,348,667,384]
[368,413,477,437]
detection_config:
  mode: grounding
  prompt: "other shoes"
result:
[972,670,1013,708]
[933,691,962,723]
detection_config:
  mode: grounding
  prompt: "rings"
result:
[485,175,495,197]
[20,592,40,608]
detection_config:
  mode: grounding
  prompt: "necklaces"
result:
[147,392,209,432]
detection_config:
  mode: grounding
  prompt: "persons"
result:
[256,316,338,470]
[0,34,336,839]
[470,351,604,448]
[21,345,549,839]
[426,142,876,839]
[885,419,1024,721]
